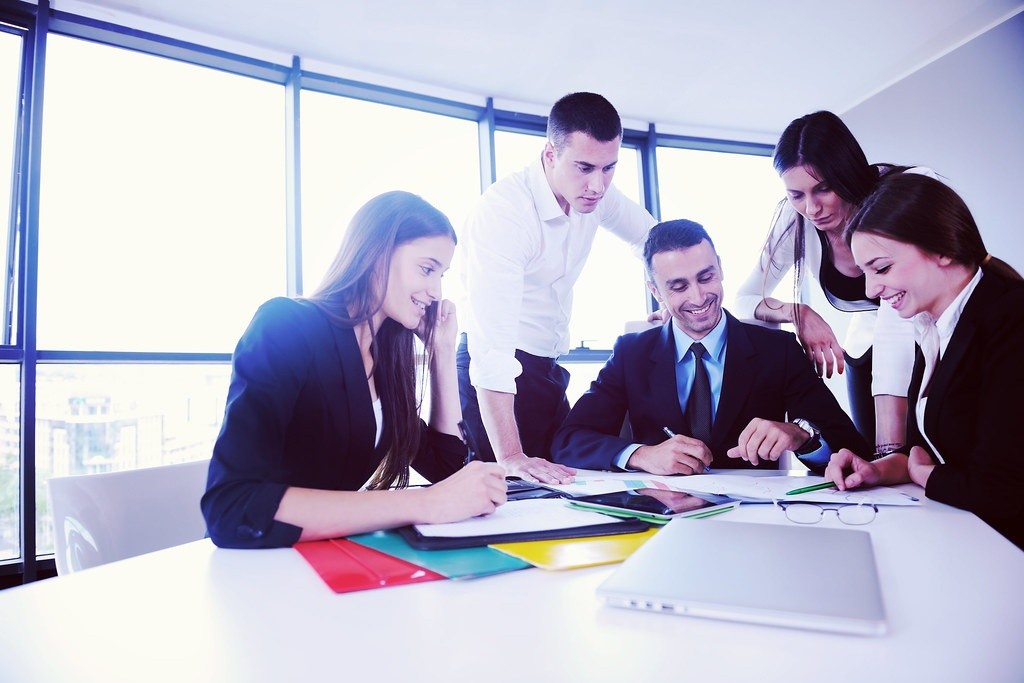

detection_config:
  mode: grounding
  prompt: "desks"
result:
[0,470,1024,683]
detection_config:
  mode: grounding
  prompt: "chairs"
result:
[46,459,212,577]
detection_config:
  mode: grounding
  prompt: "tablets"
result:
[563,486,743,521]
[595,518,888,639]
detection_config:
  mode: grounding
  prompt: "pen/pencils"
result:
[457,419,483,462]
[786,481,836,495]
[662,426,711,472]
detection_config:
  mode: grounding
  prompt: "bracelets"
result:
[873,446,907,460]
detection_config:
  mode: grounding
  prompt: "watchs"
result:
[789,418,822,452]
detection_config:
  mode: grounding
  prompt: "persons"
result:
[456,91,670,491]
[735,109,1024,550]
[198,193,509,550]
[550,219,866,477]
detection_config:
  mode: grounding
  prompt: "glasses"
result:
[775,498,879,526]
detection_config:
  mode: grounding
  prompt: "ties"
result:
[685,343,713,450]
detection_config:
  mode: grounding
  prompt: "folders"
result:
[365,476,650,552]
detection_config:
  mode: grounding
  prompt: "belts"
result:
[460,333,557,369]
